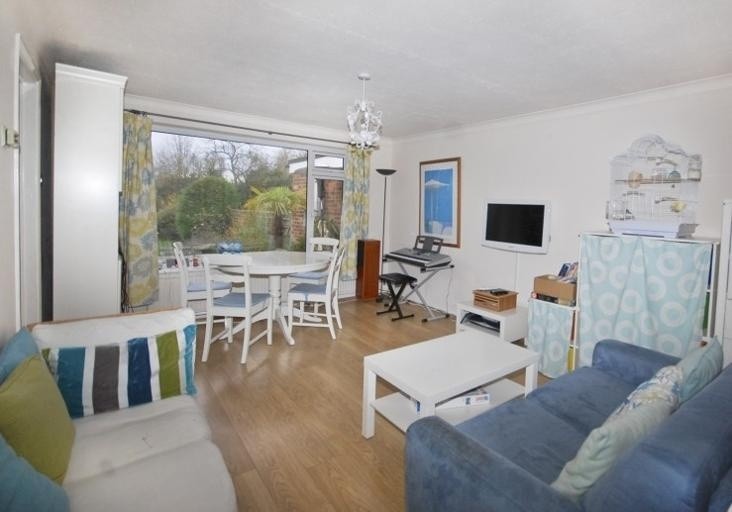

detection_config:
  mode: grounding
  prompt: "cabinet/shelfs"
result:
[570,230,721,372]
[50,61,129,322]
[525,296,577,381]
[712,199,732,372]
[456,302,528,343]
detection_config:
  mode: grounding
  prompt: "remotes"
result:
[490,291,507,296]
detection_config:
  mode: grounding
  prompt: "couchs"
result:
[0,304,242,512]
[402,338,732,512]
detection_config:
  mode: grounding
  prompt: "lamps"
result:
[346,71,383,151]
[376,167,396,300]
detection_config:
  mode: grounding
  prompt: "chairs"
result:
[286,246,345,341]
[173,241,233,344]
[286,237,340,318]
[199,253,273,365]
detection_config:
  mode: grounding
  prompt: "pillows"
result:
[0,431,72,512]
[552,400,670,497]
[609,366,684,420]
[38,323,197,421]
[677,337,723,400]
[0,353,75,482]
[0,328,38,386]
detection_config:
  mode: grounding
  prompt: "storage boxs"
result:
[534,274,577,301]
[408,386,490,414]
[472,288,519,311]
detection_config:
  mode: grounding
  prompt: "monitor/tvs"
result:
[480,200,550,254]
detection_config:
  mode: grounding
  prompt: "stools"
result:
[376,272,417,322]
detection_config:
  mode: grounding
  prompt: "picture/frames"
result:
[418,155,462,249]
